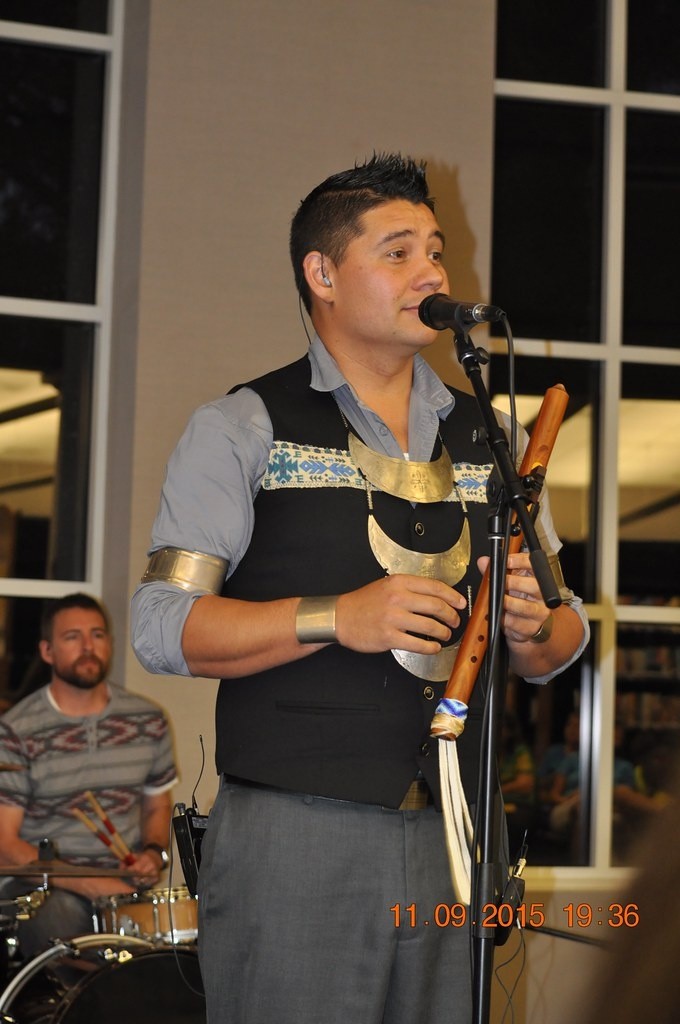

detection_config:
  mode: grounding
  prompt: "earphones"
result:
[321,272,334,287]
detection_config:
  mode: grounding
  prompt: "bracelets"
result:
[294,591,339,646]
[144,843,169,870]
[531,612,555,645]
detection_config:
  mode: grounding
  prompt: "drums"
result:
[93,884,199,944]
[0,933,207,1024]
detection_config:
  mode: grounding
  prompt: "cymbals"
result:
[0,858,156,877]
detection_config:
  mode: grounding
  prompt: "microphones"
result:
[418,293,504,330]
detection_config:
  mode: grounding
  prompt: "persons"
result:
[129,151,590,1024]
[0,593,180,962]
[494,710,668,864]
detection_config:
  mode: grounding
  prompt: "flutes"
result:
[428,380,577,746]
